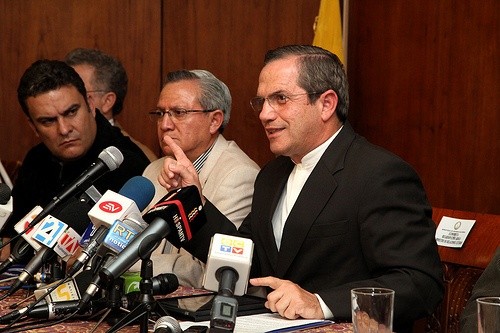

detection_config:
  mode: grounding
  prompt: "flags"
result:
[312,0,344,64]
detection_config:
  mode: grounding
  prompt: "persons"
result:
[57,69,261,288]
[66,47,158,163]
[157,43,446,326]
[0,60,151,264]
[458,243,500,333]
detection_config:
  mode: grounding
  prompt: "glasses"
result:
[250,91,326,112]
[148,105,217,122]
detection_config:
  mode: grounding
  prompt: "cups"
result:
[350,288,395,333]
[476,297,500,333]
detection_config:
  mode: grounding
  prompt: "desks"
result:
[0,279,355,333]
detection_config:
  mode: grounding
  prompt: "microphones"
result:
[27,146,125,227]
[8,174,208,318]
[209,229,253,333]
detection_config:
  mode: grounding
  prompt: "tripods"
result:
[99,250,195,332]
